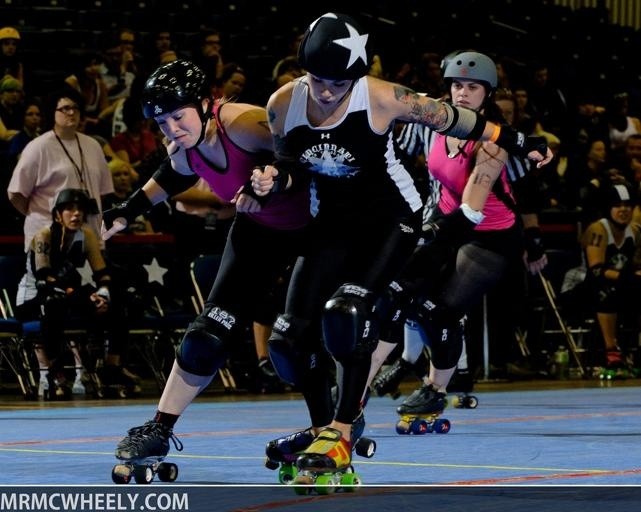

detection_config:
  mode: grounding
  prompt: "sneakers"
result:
[70,369,102,396]
[37,379,51,398]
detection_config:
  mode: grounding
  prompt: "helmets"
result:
[139,58,214,120]
[606,184,637,208]
[294,11,373,82]
[55,189,89,209]
[438,48,499,91]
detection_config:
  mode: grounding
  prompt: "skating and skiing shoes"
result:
[292,428,360,497]
[395,382,452,436]
[372,350,429,401]
[43,368,72,401]
[110,421,178,484]
[350,408,377,459]
[265,426,316,485]
[445,368,479,410]
[93,362,144,399]
[598,358,640,380]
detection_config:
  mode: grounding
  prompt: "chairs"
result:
[0,234,616,399]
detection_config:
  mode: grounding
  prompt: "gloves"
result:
[102,187,154,231]
[494,124,548,161]
[522,226,546,265]
[236,180,269,211]
[249,161,290,195]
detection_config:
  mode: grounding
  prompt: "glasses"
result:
[55,105,81,115]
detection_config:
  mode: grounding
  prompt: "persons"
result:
[249,13,557,473]
[297,50,529,417]
[96,57,463,465]
